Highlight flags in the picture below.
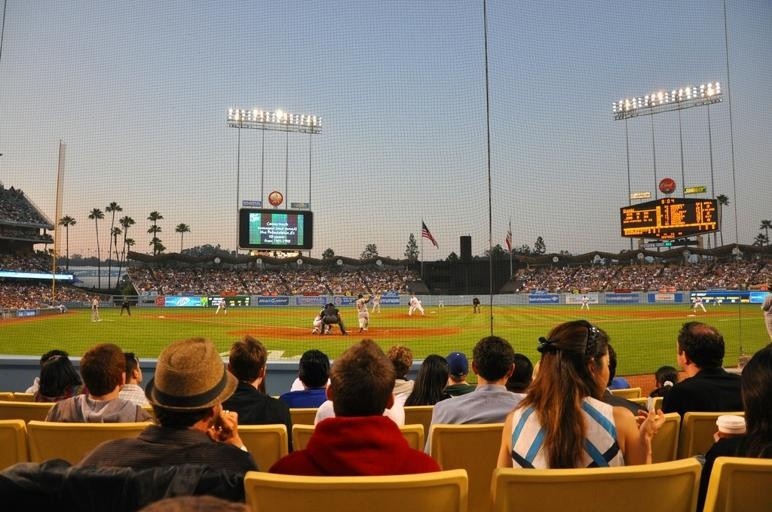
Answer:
[505,231,513,252]
[423,222,440,252]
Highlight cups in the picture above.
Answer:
[714,415,747,439]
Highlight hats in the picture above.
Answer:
[145,337,239,411]
[443,350,469,377]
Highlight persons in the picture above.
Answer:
[473,296,481,313]
[605,322,745,413]
[512,261,771,295]
[696,344,770,510]
[408,296,425,317]
[713,296,721,306]
[424,335,535,456]
[78,337,259,471]
[312,294,380,336]
[216,299,226,315]
[692,296,707,313]
[202,295,208,308]
[125,256,414,296]
[439,297,444,308]
[490,320,666,506]
[267,339,442,475]
[24,292,131,321]
[388,347,474,406]
[0,182,113,314]
[581,296,589,310]
[24,350,151,408]
[223,336,405,429]
